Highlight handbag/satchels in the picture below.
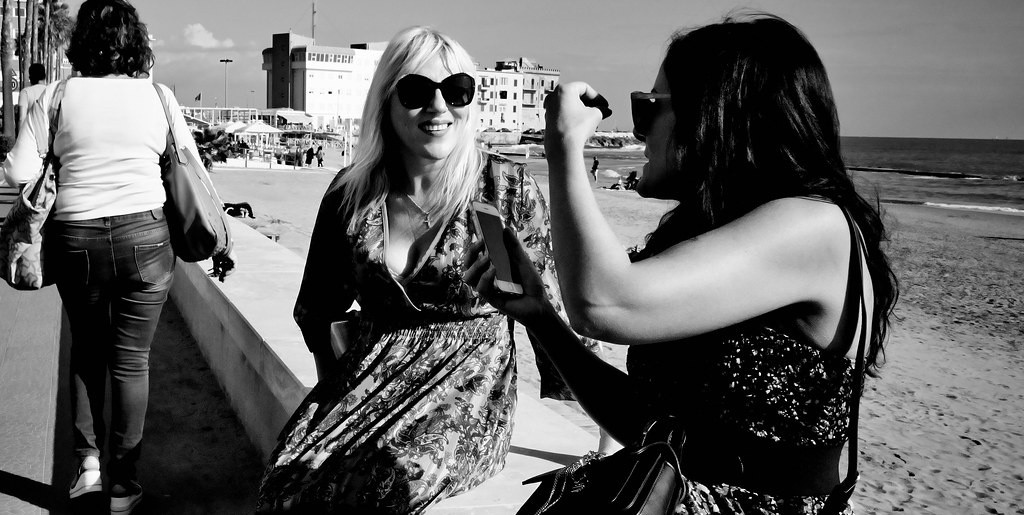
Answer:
[515,432,686,515]
[152,82,229,264]
[0,76,68,292]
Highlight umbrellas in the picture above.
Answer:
[214,120,283,155]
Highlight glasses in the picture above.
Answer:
[631,91,673,141]
[394,72,480,110]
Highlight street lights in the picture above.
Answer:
[220,59,234,109]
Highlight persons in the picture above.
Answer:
[199,137,249,172]
[592,156,599,183]
[294,146,304,169]
[305,146,327,168]
[1,0,237,515]
[17,62,48,129]
[460,15,904,515]
[626,170,637,190]
[254,24,602,515]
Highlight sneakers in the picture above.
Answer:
[110,481,143,515]
[70,455,102,499]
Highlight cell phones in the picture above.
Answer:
[469,200,525,297]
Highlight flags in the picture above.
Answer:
[195,93,201,102]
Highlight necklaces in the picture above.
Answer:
[404,194,441,230]
